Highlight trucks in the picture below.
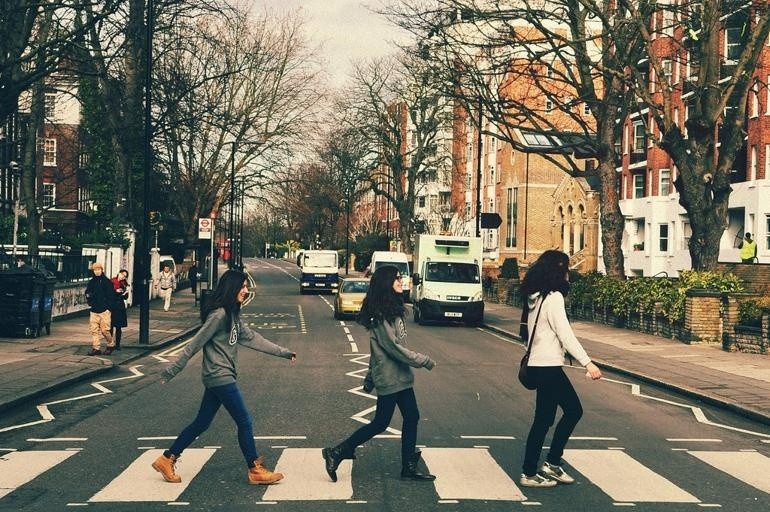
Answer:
[411,234,485,326]
[297,250,340,294]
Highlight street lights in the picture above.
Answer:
[10,161,21,265]
[445,79,484,238]
[221,139,265,270]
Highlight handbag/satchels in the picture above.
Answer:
[518,351,537,390]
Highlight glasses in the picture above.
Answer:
[396,275,402,281]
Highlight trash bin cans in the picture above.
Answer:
[41,272,56,335]
[0,265,46,338]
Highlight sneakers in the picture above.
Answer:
[104,346,116,354]
[520,473,557,487]
[88,349,102,356]
[539,461,574,484]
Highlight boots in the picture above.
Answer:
[152,450,182,482]
[401,451,436,481]
[322,441,356,482]
[248,460,284,485]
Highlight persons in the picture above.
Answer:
[84,263,117,356]
[738,232,757,264]
[633,244,641,251]
[188,262,198,293]
[154,265,176,312]
[322,263,436,481]
[152,269,297,485]
[520,251,602,487]
[108,269,130,351]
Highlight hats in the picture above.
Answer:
[92,263,102,269]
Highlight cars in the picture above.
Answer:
[335,278,372,320]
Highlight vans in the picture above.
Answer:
[369,251,410,303]
